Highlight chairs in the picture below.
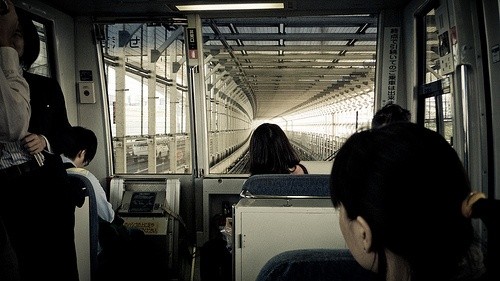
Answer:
[256,248,373,281]
[64,174,98,281]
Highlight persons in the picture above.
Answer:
[0,0,144,281]
[329,119,500,281]
[248,122,308,175]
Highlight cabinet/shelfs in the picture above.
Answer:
[233,198,351,281]
